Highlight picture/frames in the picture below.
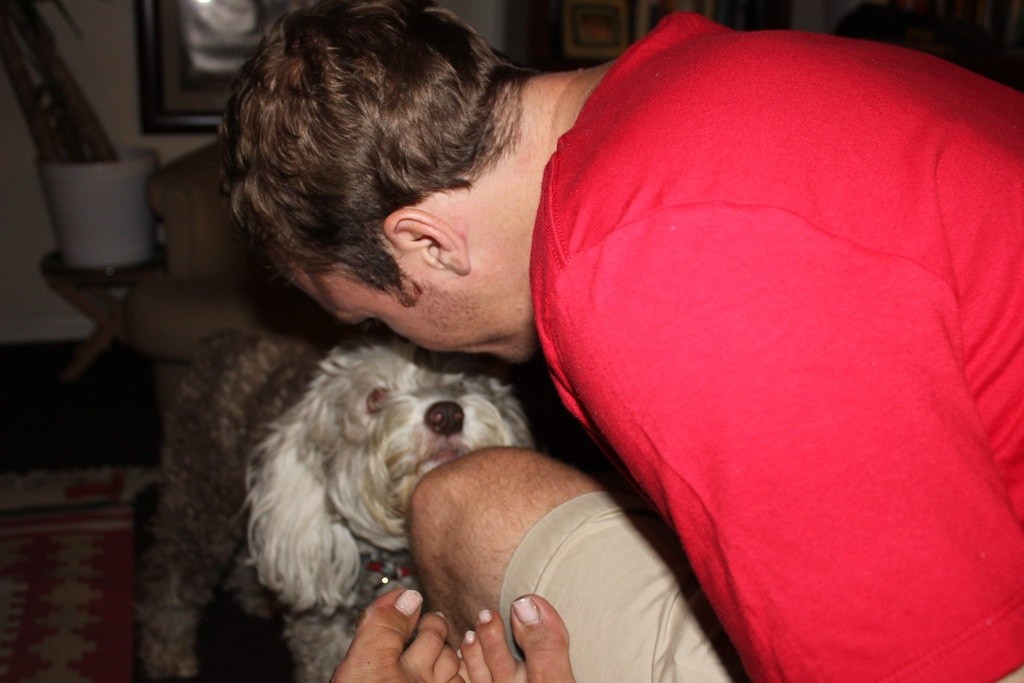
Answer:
[525,0,793,72]
[131,0,288,137]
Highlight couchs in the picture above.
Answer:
[120,143,509,416]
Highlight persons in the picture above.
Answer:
[219,0,1024,683]
[329,586,573,683]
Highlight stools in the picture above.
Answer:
[40,242,170,382]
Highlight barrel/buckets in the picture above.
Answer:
[37,142,159,270]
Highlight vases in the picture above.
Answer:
[34,140,160,269]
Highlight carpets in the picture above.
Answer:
[0,457,154,683]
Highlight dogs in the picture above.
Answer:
[131,321,537,683]
